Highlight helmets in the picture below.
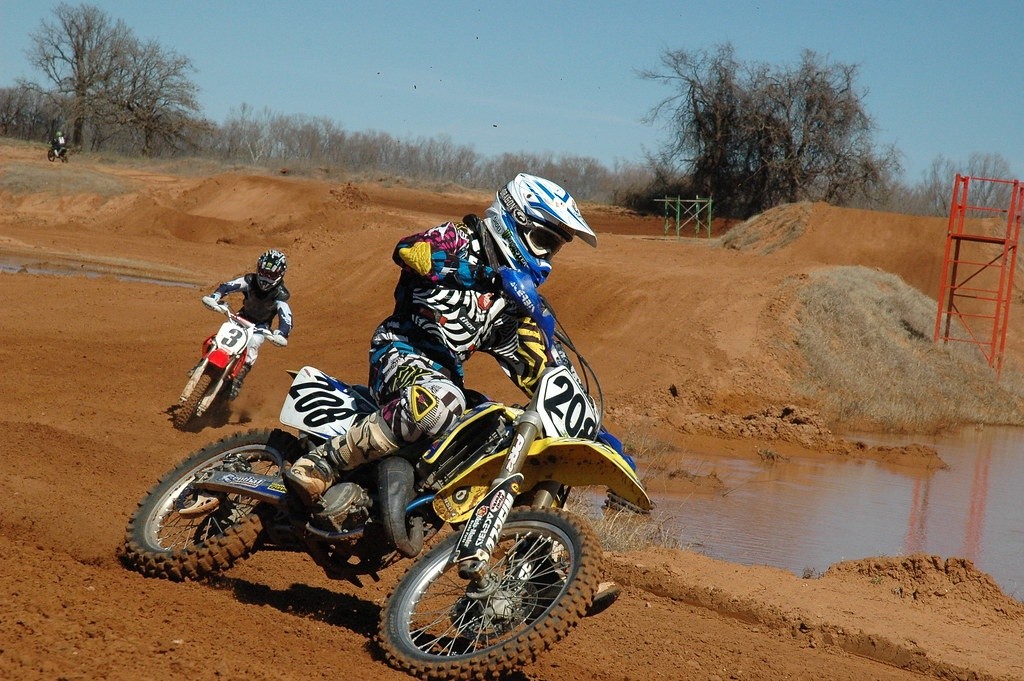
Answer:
[56,132,62,136]
[485,173,597,288]
[257,249,287,290]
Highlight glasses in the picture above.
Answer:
[501,187,568,263]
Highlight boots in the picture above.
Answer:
[286,409,401,501]
[187,334,218,378]
[223,362,251,401]
[520,500,621,624]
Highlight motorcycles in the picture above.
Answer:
[46,139,69,165]
[121,269,655,681]
[173,296,288,432]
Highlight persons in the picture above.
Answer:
[48,131,66,158]
[286,173,610,505]
[186,249,295,401]
